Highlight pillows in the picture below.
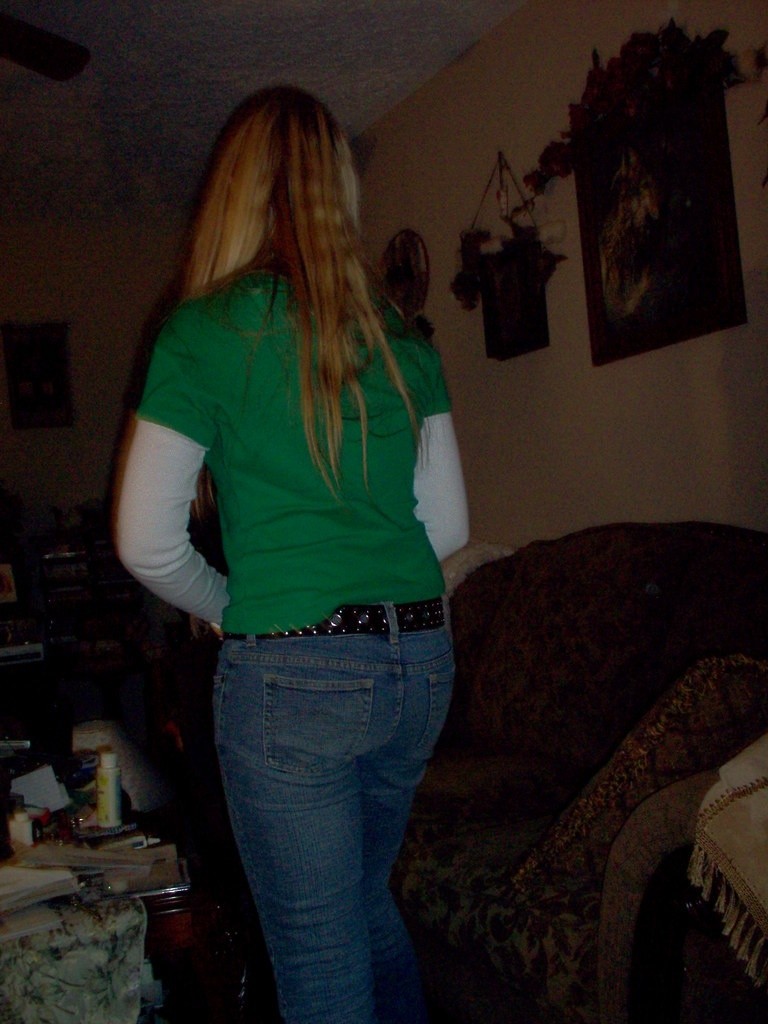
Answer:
[509,649,768,895]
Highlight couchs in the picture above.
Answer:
[388,520,768,1024]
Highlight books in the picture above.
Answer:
[1,840,195,946]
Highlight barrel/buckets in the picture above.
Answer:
[142,887,210,981]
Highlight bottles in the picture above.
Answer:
[97,751,122,828]
[9,809,34,846]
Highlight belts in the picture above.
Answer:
[222,598,445,641]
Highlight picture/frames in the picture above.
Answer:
[567,84,749,365]
[478,247,553,359]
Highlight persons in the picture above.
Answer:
[112,81,475,1024]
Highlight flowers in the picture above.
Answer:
[522,8,748,195]
